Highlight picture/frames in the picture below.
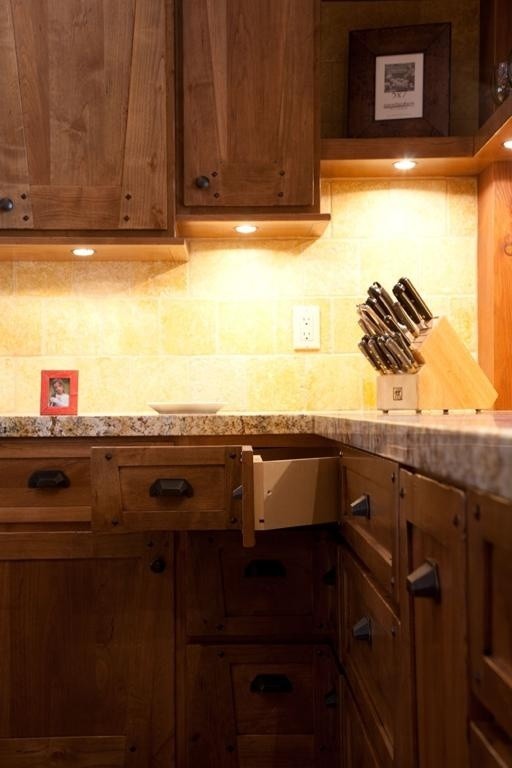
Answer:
[343,21,453,143]
[39,370,78,416]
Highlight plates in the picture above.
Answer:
[146,400,226,415]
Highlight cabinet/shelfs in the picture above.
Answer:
[1,0,191,263]
[177,1,332,243]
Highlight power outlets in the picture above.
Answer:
[292,304,321,351]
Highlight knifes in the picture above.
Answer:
[355,276,434,374]
